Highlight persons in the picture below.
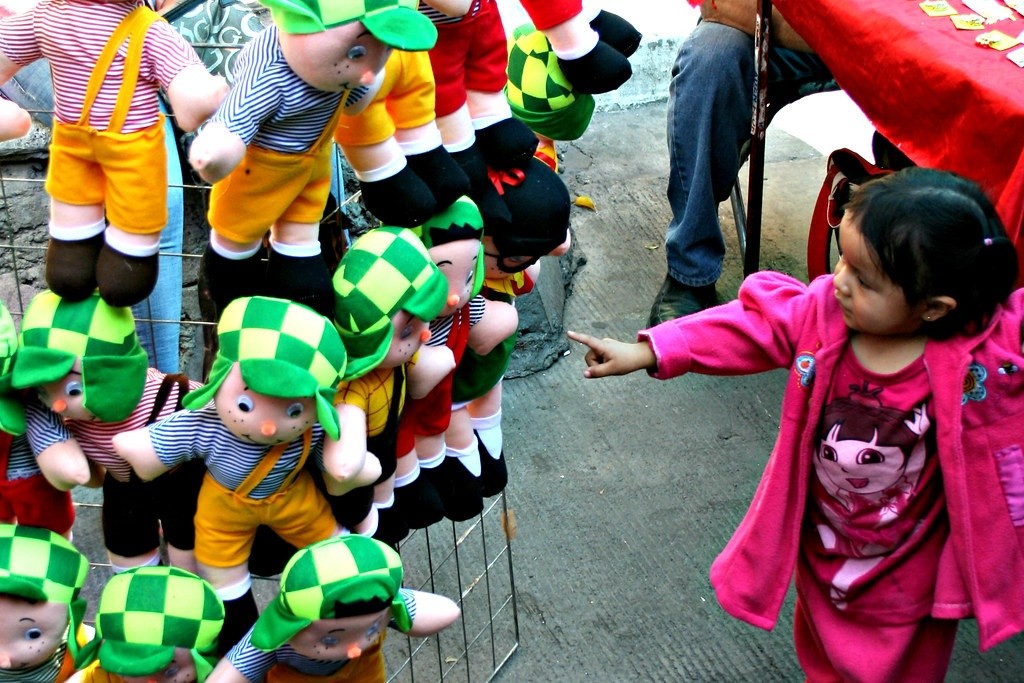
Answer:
[568,168,1024,683]
[646,0,910,328]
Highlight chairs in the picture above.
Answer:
[685,0,842,262]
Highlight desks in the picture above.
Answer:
[744,0,1024,278]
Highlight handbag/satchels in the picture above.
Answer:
[807,148,895,283]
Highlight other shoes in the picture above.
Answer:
[646,273,721,329]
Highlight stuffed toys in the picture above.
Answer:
[0,0,645,683]
